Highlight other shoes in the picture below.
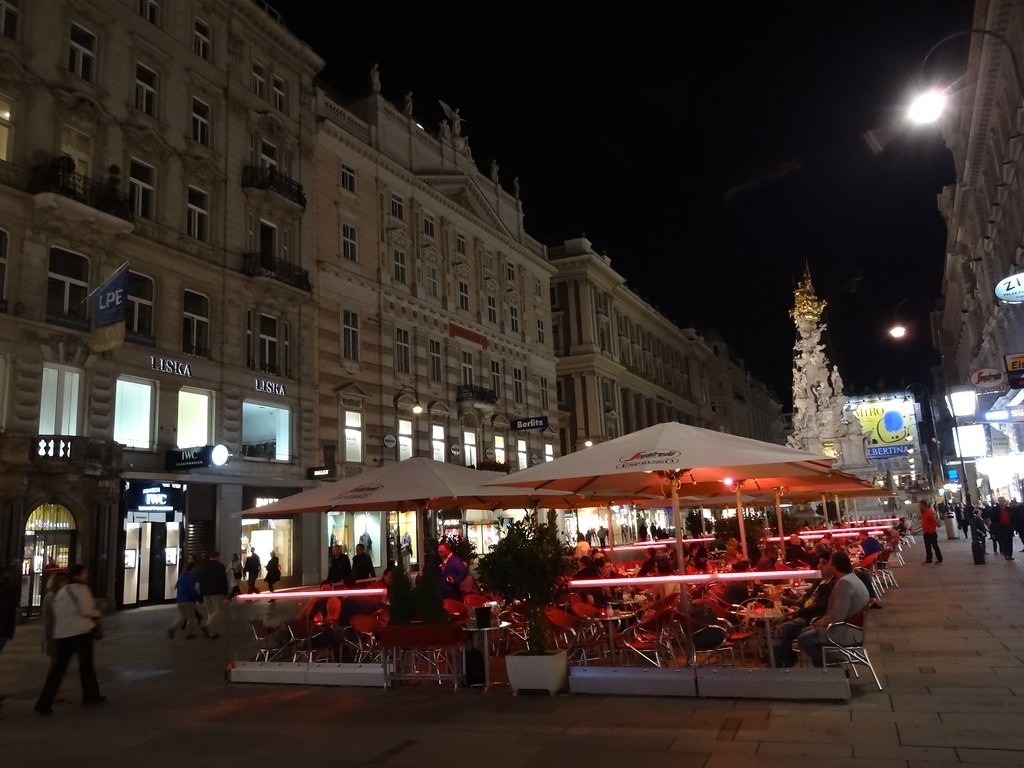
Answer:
[83,696,106,702]
[166,628,175,639]
[185,634,196,639]
[1005,556,1015,560]
[995,551,998,555]
[221,598,229,603]
[922,561,932,563]
[1019,549,1024,552]
[35,703,54,713]
[935,558,943,563]
[984,551,989,554]
[199,625,221,640]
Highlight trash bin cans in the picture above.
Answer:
[944,511,959,539]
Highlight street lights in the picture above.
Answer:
[906,423,941,526]
[572,428,592,533]
[902,382,948,506]
[395,386,422,570]
[889,297,972,508]
[907,27,1024,124]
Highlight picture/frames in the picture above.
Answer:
[124,549,137,569]
[165,546,177,566]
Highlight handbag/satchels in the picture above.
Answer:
[234,572,242,579]
[92,619,103,640]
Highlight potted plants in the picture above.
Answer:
[471,495,578,697]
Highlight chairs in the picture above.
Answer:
[250,526,916,691]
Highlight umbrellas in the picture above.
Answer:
[232,456,586,574]
[583,468,899,588]
[478,421,839,667]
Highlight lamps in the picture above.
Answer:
[571,428,593,447]
[393,386,422,415]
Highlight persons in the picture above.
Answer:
[34,557,105,716]
[167,552,227,641]
[359,531,372,554]
[560,510,912,640]
[0,568,17,702]
[768,551,871,678]
[919,497,1024,564]
[786,262,863,450]
[295,543,469,663]
[370,63,519,200]
[222,547,279,604]
[401,531,412,546]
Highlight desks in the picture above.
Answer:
[609,599,641,633]
[774,582,813,591]
[594,611,635,666]
[462,622,514,694]
[736,608,795,667]
[371,617,470,691]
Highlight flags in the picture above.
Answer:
[91,258,130,352]
